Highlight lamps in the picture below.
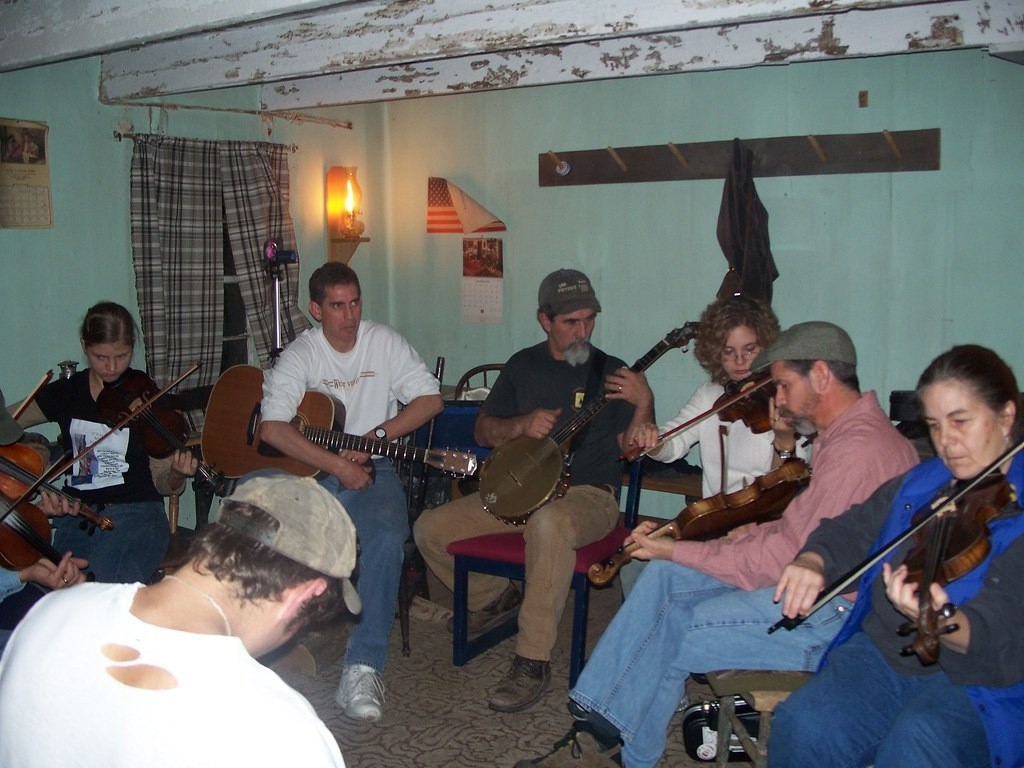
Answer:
[326,165,370,266]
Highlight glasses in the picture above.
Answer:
[721,351,760,361]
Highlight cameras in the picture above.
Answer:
[263,238,297,267]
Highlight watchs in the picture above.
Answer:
[373,426,388,440]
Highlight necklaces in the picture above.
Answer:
[158,571,232,636]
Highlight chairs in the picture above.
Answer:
[396,355,445,657]
[447,456,643,690]
[707,670,813,768]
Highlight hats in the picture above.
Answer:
[0,389,23,445]
[750,321,855,373]
[538,268,601,316]
[214,474,363,615]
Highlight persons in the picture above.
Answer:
[6,302,205,583]
[0,490,87,604]
[259,263,445,723]
[506,321,921,768]
[767,343,1024,768]
[0,475,363,768]
[413,269,657,715]
[620,295,813,603]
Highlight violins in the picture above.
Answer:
[95,369,228,497]
[0,443,114,532]
[893,466,1015,667]
[0,496,97,584]
[587,457,814,586]
[712,373,818,447]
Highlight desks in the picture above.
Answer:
[451,462,700,501]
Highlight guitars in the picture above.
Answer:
[479,320,704,526]
[201,365,480,481]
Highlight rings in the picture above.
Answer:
[64,579,68,583]
[773,418,780,422]
[619,386,623,392]
[351,457,359,462]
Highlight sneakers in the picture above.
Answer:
[516,723,622,768]
[447,581,522,635]
[334,664,386,722]
[488,657,550,711]
